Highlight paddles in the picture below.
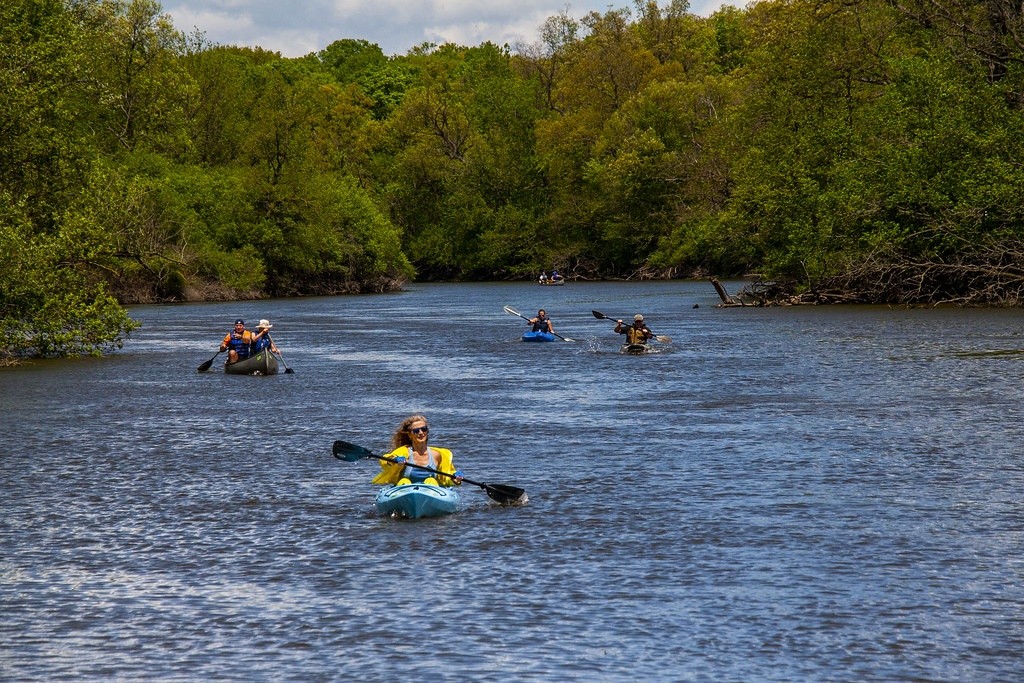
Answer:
[265,328,295,374]
[588,308,672,343]
[198,346,227,372]
[501,302,575,343]
[332,438,530,509]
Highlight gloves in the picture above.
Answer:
[394,457,406,464]
[453,471,465,480]
[551,331,554,333]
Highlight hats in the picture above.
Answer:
[235,319,244,325]
[633,313,644,321]
[553,271,557,276]
[255,319,273,328]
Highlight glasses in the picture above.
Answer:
[411,426,427,434]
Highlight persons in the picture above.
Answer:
[613,313,654,348]
[527,309,555,334]
[252,319,280,355]
[551,270,563,282]
[371,414,465,488]
[540,271,549,281]
[220,318,252,364]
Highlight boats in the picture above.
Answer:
[620,343,652,354]
[539,278,565,286]
[224,347,280,375]
[522,331,554,342]
[375,482,461,518]
